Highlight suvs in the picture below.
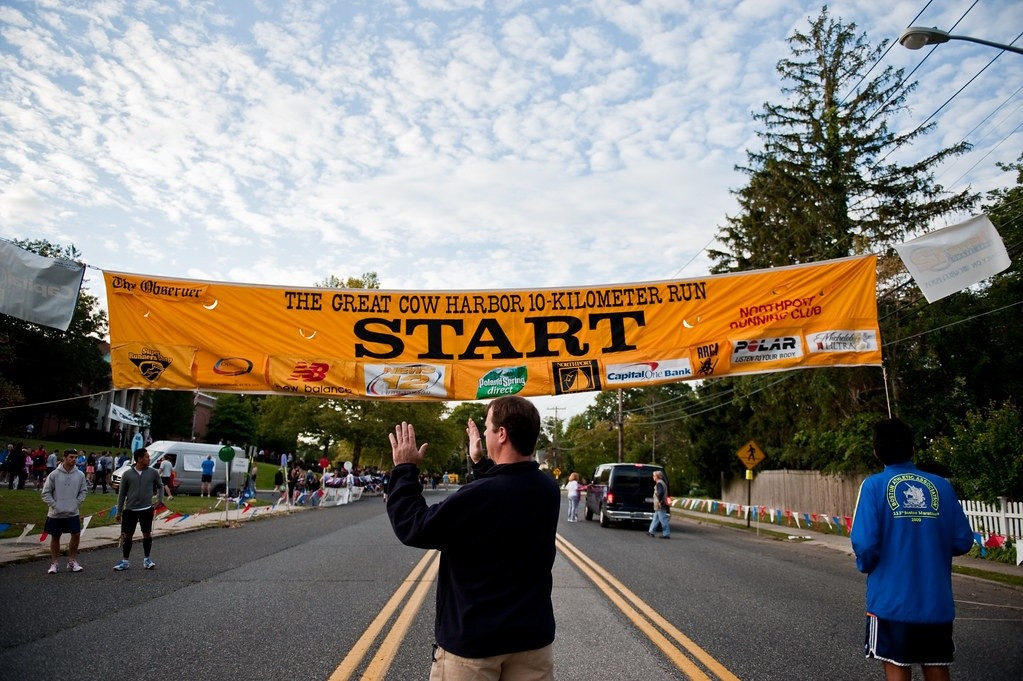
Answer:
[583,463,670,529]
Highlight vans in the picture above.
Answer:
[109,441,246,497]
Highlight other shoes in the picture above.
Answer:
[646,533,655,537]
[660,535,671,539]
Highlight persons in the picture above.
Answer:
[646,470,672,539]
[40,449,88,574]
[564,473,578,523]
[852,417,974,681]
[575,477,593,519]
[113,449,164,570]
[386,395,563,681]
[0,436,453,508]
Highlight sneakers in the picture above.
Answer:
[114,560,129,571]
[48,563,60,573]
[67,561,83,571]
[143,559,155,569]
